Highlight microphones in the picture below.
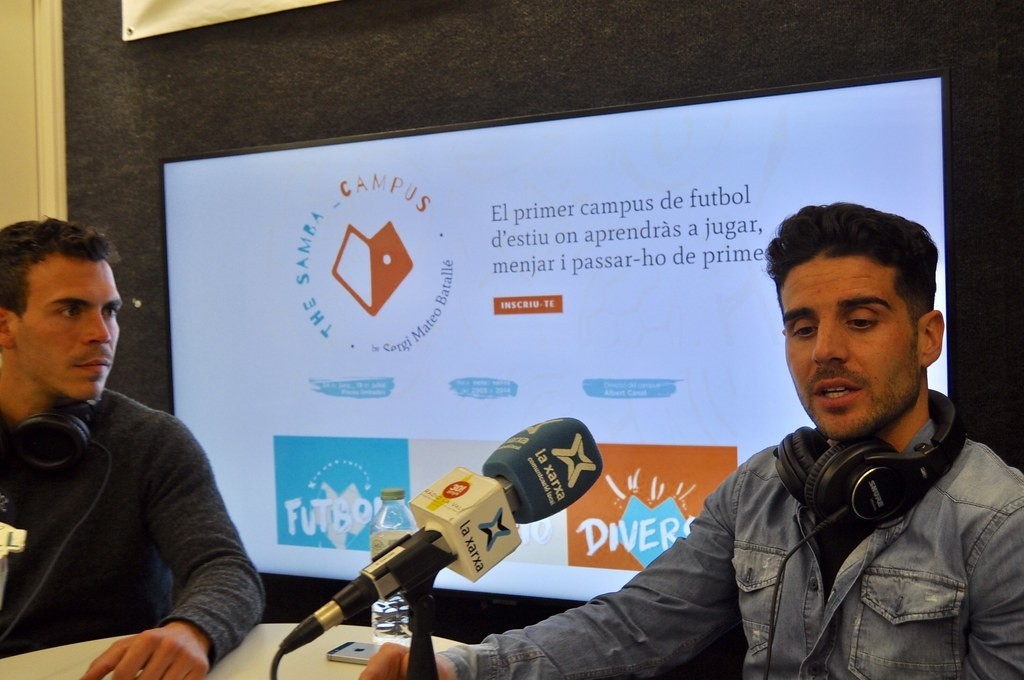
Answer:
[280,418,603,654]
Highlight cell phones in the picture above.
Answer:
[327,642,382,664]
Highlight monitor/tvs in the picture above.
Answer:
[157,66,951,613]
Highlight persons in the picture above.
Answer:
[0,218,265,680]
[359,203,1024,680]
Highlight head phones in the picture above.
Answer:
[0,398,102,482]
[772,388,967,534]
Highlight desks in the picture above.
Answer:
[0,622,464,680]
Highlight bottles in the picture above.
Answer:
[368,488,414,651]
